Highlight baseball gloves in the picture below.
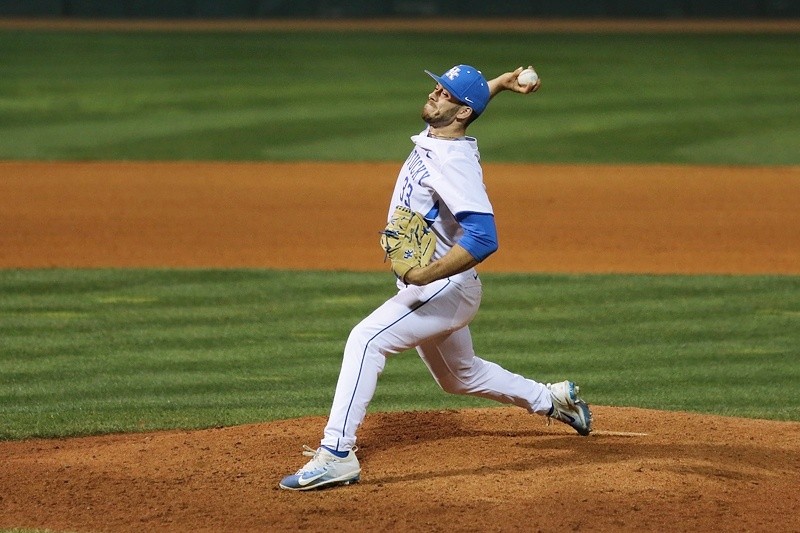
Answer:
[378,203,438,289]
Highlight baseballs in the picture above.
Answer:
[517,68,539,87]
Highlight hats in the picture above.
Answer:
[424,64,491,116]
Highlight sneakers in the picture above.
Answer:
[546,379,593,436]
[279,444,362,490]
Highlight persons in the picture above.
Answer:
[278,65,594,492]
